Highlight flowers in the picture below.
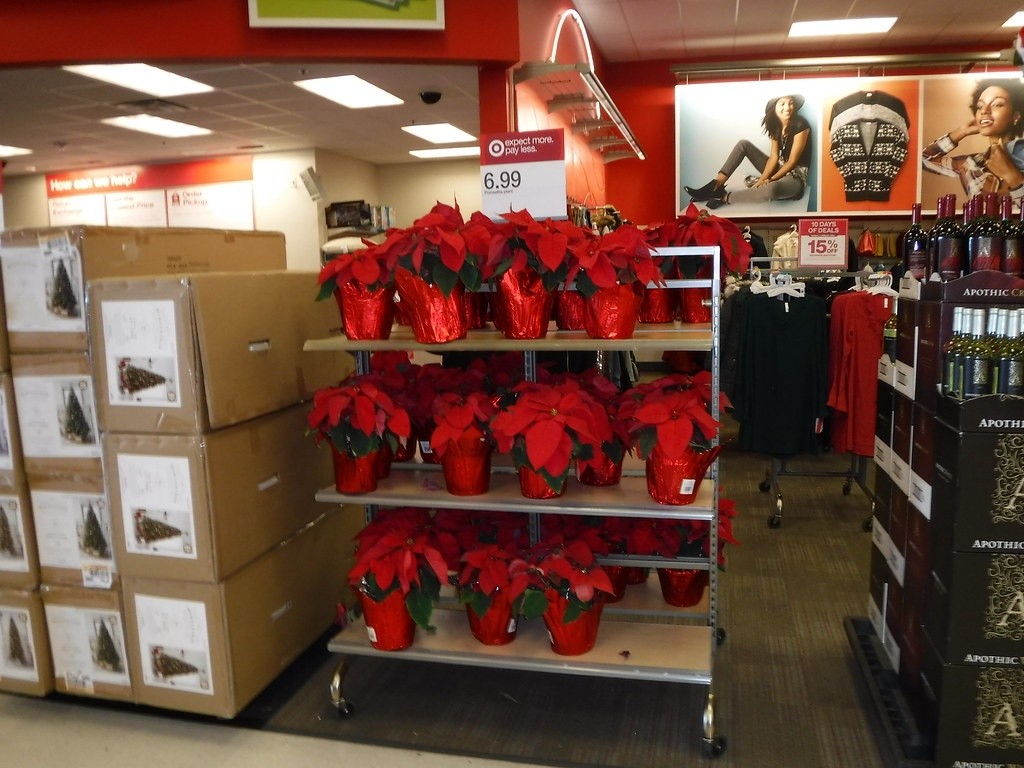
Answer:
[307,192,743,628]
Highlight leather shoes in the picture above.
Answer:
[684,180,728,209]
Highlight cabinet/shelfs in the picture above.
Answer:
[301,244,730,758]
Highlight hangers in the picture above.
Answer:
[731,224,899,297]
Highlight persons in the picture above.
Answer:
[684,94,810,208]
[923,78,1024,210]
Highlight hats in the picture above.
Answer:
[765,89,805,112]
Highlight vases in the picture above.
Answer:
[331,268,709,656]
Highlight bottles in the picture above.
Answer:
[940,304,1024,400]
[903,188,1024,282]
[883,294,900,367]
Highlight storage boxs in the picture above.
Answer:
[0,199,395,719]
[867,270,1024,768]
